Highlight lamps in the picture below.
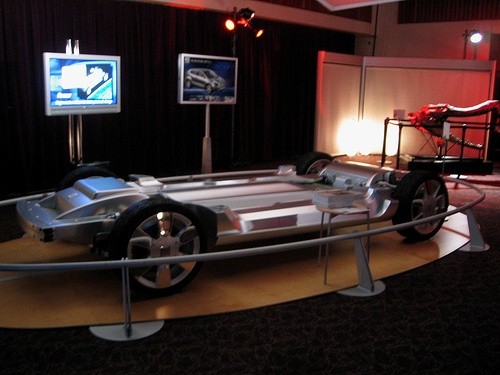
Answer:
[224,7,264,37]
[463,29,482,59]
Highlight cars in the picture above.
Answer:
[185,68,225,95]
